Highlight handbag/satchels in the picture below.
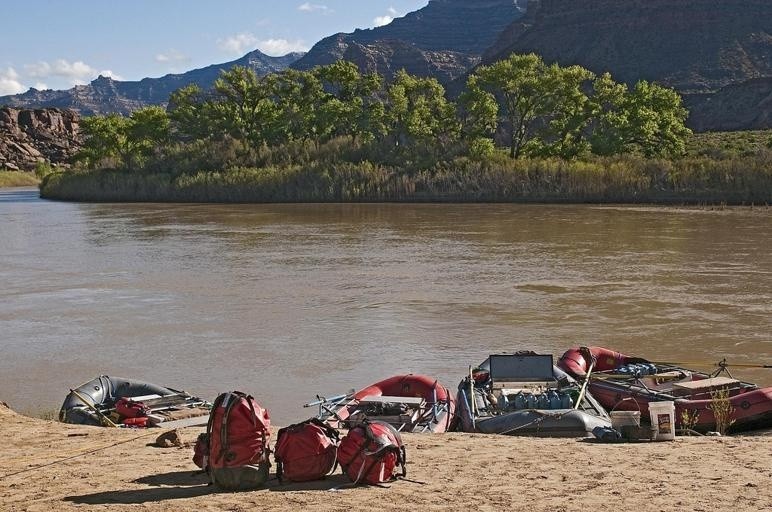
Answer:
[338,421,402,484]
[274,418,338,482]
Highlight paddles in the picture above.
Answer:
[469,365,476,430]
[303,389,355,408]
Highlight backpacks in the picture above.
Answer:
[204,391,270,491]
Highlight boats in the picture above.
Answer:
[459,352,612,438]
[316,373,455,435]
[58,374,212,430]
[558,346,772,432]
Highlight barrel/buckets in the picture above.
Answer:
[609,396,641,440]
[649,400,676,441]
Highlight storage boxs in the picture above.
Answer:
[673,377,740,399]
